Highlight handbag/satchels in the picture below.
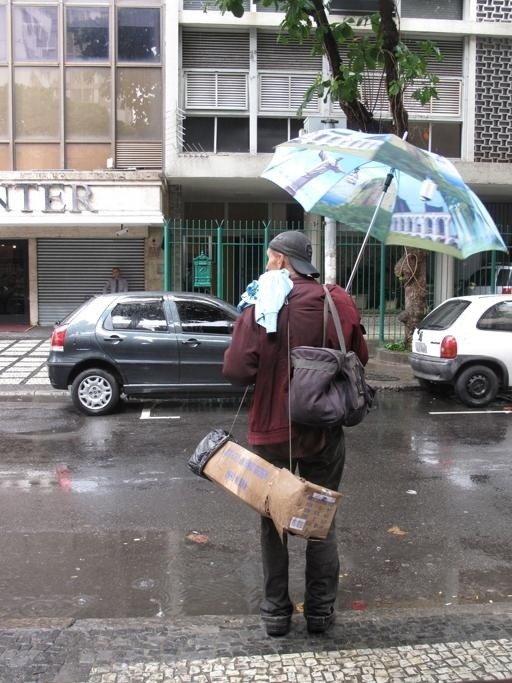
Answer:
[288,279,368,427]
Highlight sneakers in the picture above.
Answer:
[262,613,335,636]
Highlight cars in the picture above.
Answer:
[407,262,512,408]
[46,291,255,416]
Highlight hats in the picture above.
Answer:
[268,230,321,282]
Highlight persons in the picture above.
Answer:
[222,228,370,639]
[102,266,129,294]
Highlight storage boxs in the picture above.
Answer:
[203,441,343,545]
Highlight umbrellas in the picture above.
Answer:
[260,125,512,297]
